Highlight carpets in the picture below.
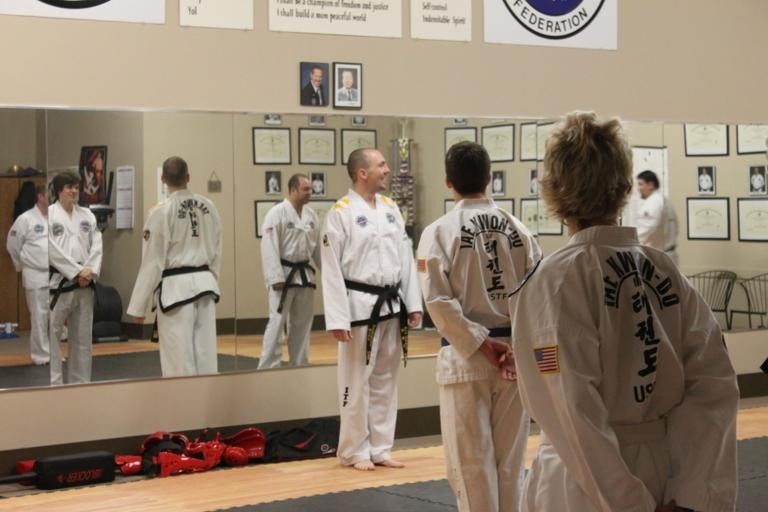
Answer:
[0,349,289,389]
[201,435,767,512]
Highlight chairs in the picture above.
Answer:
[727,273,766,330]
[685,269,735,330]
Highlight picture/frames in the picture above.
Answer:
[242,118,382,241]
[677,123,765,244]
[331,62,363,110]
[436,120,566,240]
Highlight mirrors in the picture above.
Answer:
[0,107,765,393]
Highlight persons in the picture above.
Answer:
[318,144,426,471]
[312,174,323,194]
[751,168,765,193]
[493,172,504,194]
[635,171,680,271]
[336,68,357,101]
[6,185,66,367]
[300,65,324,106]
[508,108,740,511]
[414,141,547,512]
[530,171,536,192]
[256,174,319,370]
[47,170,104,389]
[698,168,713,192]
[124,154,224,377]
[268,172,279,193]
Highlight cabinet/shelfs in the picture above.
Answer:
[0,169,46,331]
